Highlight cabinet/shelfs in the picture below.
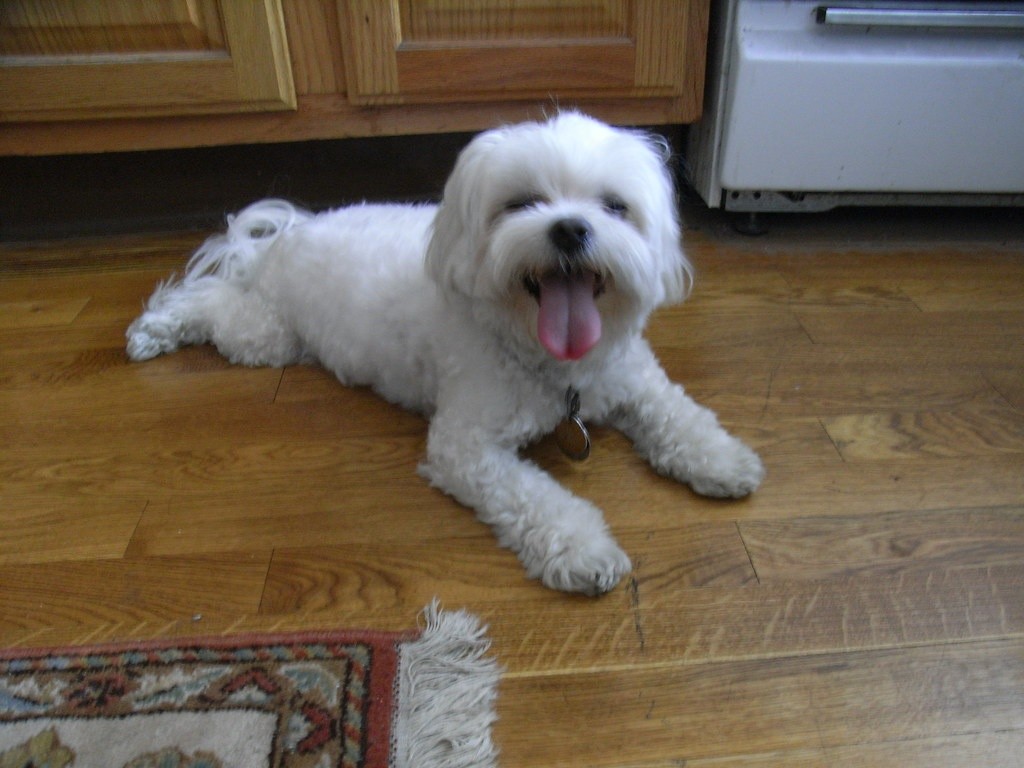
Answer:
[0,0,712,157]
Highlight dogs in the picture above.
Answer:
[125,91,763,598]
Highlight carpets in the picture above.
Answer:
[0,590,507,768]
